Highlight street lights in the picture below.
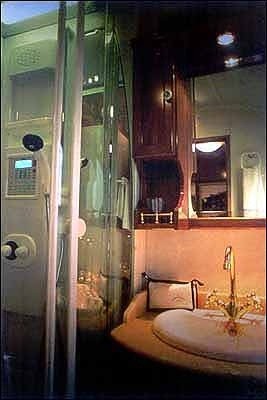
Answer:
[141,272,204,311]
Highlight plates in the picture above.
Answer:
[132,63,189,229]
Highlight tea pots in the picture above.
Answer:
[189,51,265,228]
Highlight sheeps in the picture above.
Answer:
[222,245,239,319]
